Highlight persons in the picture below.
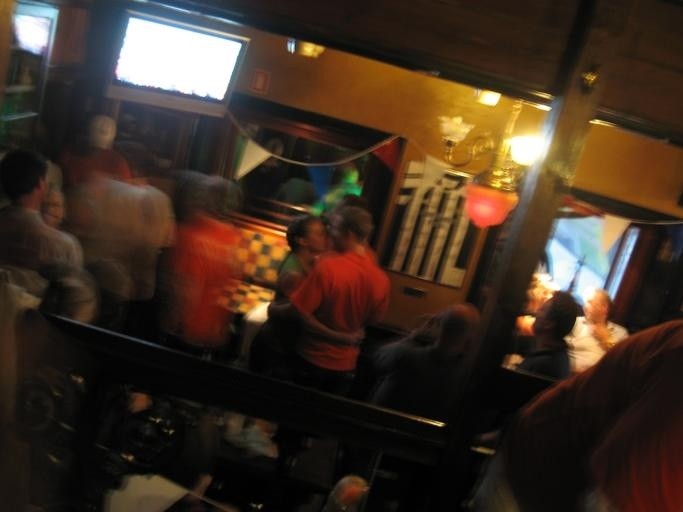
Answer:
[504,317,682,512]
[0,114,632,509]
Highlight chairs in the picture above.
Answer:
[209,215,291,355]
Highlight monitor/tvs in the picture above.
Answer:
[219,108,380,235]
[103,7,251,119]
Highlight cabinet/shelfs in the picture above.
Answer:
[0,1,58,153]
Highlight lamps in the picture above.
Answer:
[439,74,558,230]
